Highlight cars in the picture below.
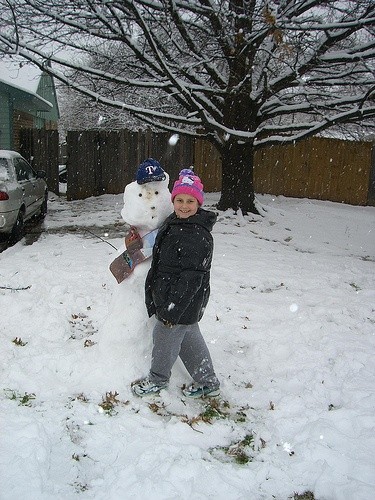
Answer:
[0,149,49,238]
[58,156,67,182]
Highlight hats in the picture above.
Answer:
[171,169,205,205]
[135,159,166,185]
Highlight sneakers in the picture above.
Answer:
[183,382,220,398]
[131,373,171,396]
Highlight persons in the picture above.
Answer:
[125,169,221,399]
